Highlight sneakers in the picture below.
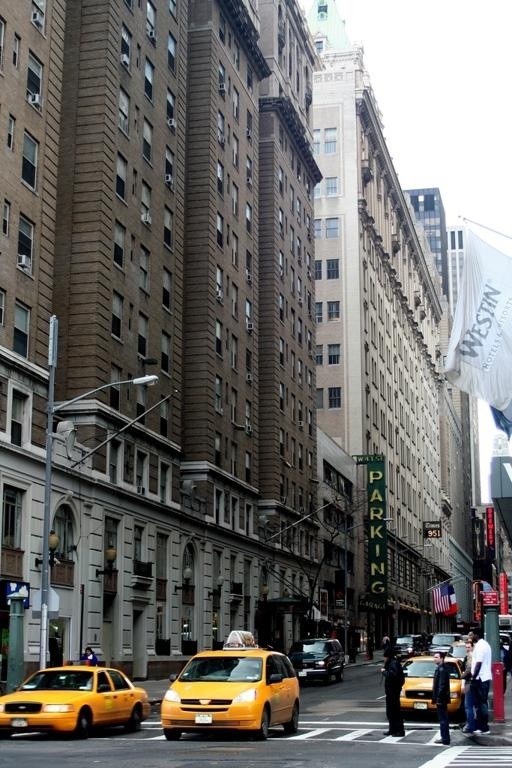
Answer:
[459,723,490,735]
[435,738,450,744]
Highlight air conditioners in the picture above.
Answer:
[146,28,156,40]
[167,117,177,131]
[18,254,30,270]
[219,82,226,93]
[219,135,227,145]
[29,93,43,109]
[32,12,44,28]
[122,54,132,67]
[166,174,173,184]
[141,214,153,227]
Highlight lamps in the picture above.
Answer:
[260,584,269,602]
[34,530,224,598]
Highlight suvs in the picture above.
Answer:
[287,637,346,685]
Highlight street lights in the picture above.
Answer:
[38,312,162,670]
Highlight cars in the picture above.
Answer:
[395,633,462,661]
[399,655,471,721]
[1,664,153,739]
[160,630,301,741]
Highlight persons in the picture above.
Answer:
[80,646,98,666]
[379,647,406,737]
[382,633,391,645]
[457,640,477,733]
[465,627,493,736]
[430,651,450,745]
[498,635,512,695]
[365,636,374,660]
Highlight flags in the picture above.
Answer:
[440,584,458,618]
[442,227,511,438]
[431,583,450,614]
